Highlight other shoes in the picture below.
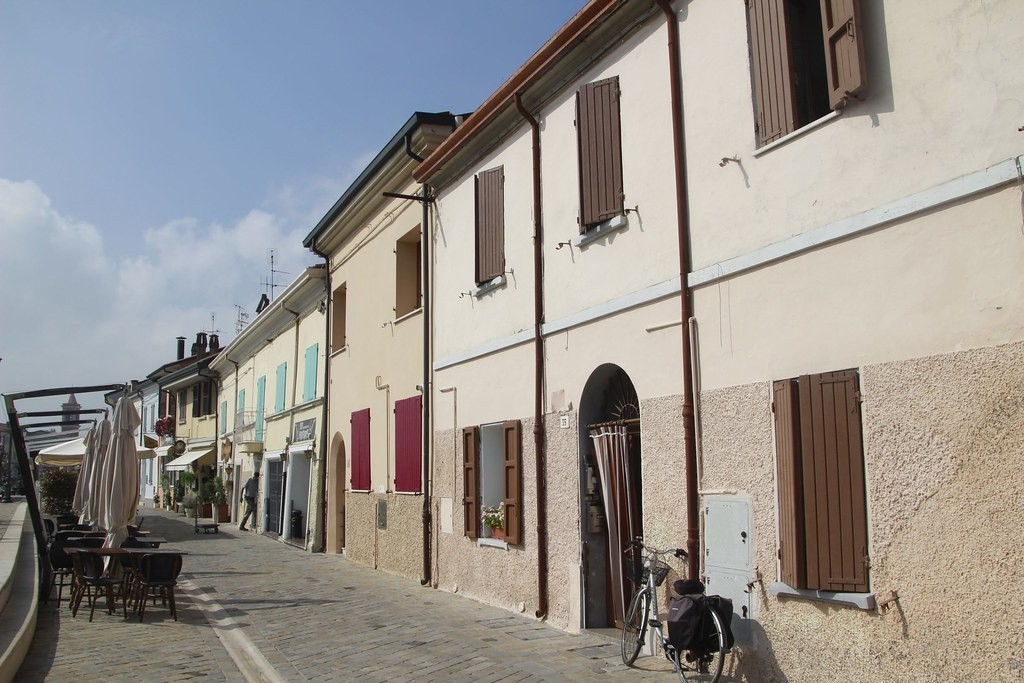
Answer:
[239,526,249,531]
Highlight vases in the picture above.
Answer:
[490,526,505,537]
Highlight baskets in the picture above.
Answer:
[625,555,671,586]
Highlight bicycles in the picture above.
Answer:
[620,535,730,683]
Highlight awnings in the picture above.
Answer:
[156,445,174,456]
[165,447,216,471]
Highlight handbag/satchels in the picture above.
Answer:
[667,594,735,653]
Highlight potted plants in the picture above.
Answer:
[180,489,196,517]
[198,480,213,517]
[165,491,172,511]
[212,477,229,524]
[153,494,159,508]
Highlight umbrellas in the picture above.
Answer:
[35,382,157,579]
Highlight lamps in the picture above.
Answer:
[225,463,234,475]
[279,441,292,461]
[305,438,317,460]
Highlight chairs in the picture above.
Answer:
[44,512,186,623]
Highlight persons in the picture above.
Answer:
[239,472,259,531]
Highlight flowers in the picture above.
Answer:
[479,501,504,527]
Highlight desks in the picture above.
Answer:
[60,530,107,536]
[124,546,189,612]
[125,536,168,548]
[63,548,129,610]
[135,530,151,536]
[66,535,105,548]
